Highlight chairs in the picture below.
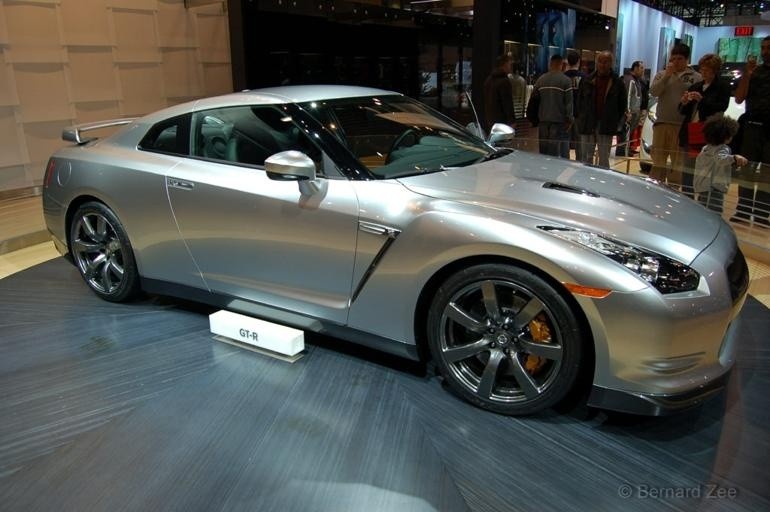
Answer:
[228,115,319,168]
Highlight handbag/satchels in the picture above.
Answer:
[526,87,541,127]
[687,122,707,158]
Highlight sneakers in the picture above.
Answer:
[615,149,639,157]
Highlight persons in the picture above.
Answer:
[728,35,770,230]
[478,55,519,130]
[529,43,754,214]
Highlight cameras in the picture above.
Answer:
[688,92,692,100]
